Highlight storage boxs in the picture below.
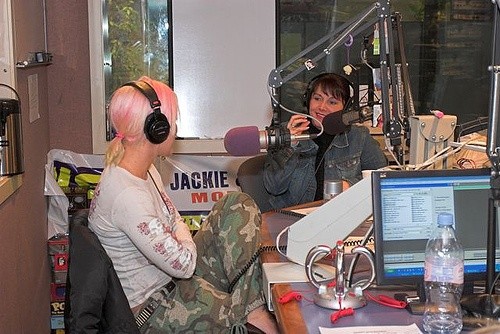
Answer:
[49,238,68,334]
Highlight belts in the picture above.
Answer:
[135,281,175,328]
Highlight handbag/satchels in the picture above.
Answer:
[409,114,457,170]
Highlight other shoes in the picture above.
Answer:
[238,321,265,334]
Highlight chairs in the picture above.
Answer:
[237,154,272,213]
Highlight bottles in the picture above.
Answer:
[422,213,464,334]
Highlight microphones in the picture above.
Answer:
[322,106,371,136]
[223,126,318,156]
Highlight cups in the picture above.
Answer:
[324,180,343,203]
[362,170,377,179]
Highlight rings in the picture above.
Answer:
[291,124,294,128]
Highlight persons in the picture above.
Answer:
[87,76,281,334]
[263,72,388,210]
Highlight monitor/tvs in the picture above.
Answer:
[371,167,500,316]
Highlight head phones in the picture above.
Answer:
[301,72,359,112]
[121,80,170,144]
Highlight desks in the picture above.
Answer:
[259,200,500,334]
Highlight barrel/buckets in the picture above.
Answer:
[0,83,24,175]
